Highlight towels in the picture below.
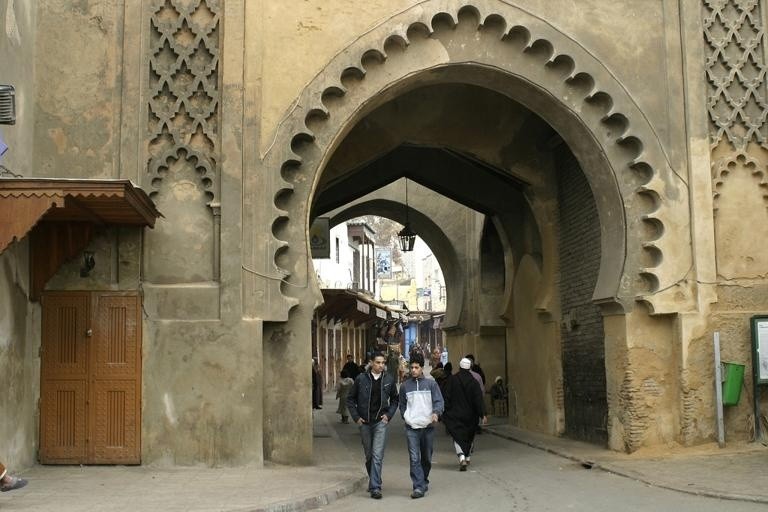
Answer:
[720,361,745,406]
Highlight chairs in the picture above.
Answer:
[410,490,425,498]
[369,490,383,498]
[459,455,472,471]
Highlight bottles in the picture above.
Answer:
[459,357,472,371]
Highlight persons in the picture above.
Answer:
[400,357,444,499]
[442,358,487,472]
[312,341,508,433]
[0,463,28,493]
[348,354,399,500]
